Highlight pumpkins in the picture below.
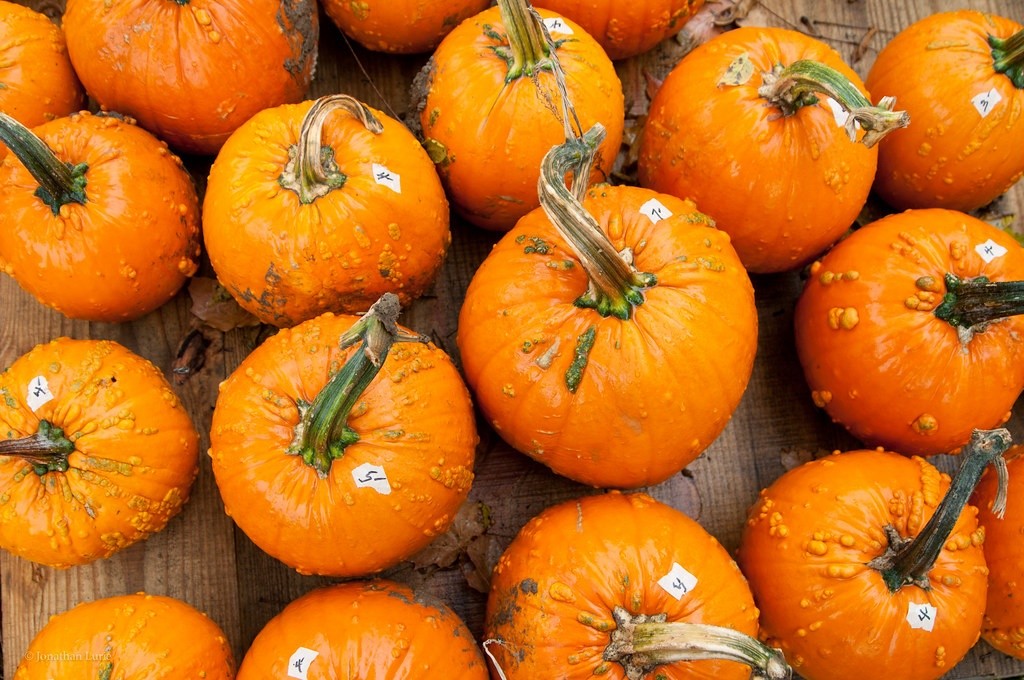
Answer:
[0,0,1024,680]
